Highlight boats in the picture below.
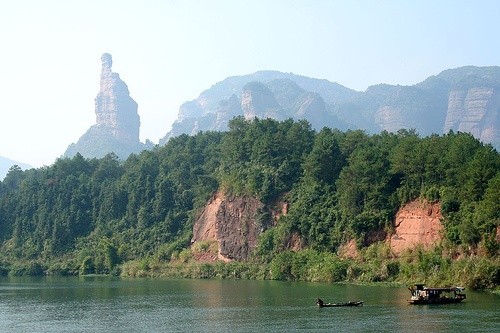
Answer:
[405,282,466,305]
[316,297,364,308]
